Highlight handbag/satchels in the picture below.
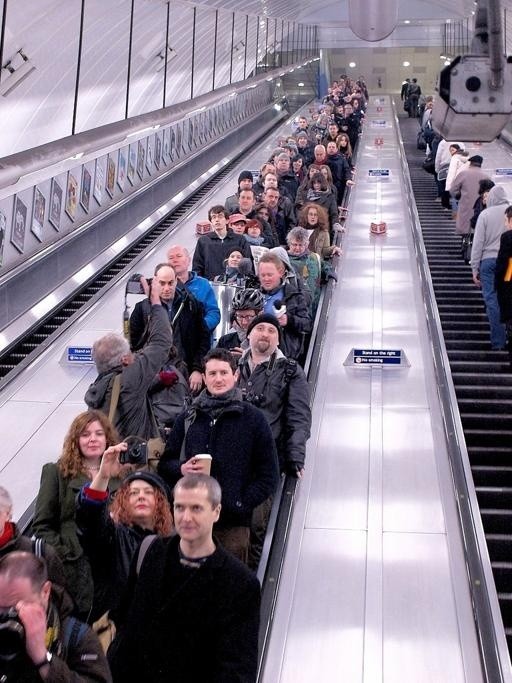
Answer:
[422,154,435,174]
[461,224,471,263]
[416,132,427,150]
[404,101,410,111]
[108,430,166,471]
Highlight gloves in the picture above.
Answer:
[325,271,338,282]
[159,370,178,387]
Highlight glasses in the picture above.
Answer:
[237,314,254,320]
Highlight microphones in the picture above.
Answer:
[272,299,287,331]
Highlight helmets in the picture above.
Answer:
[232,288,264,310]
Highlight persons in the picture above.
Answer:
[83,185,344,474]
[225,75,368,234]
[0,349,278,682]
[401,76,511,372]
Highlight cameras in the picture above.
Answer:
[118,444,148,464]
[126,274,152,294]
[0,607,26,661]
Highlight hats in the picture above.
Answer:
[238,171,253,185]
[228,214,247,225]
[246,314,282,339]
[297,131,308,141]
[468,156,482,163]
[124,470,168,496]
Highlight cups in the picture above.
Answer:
[193,452,212,479]
